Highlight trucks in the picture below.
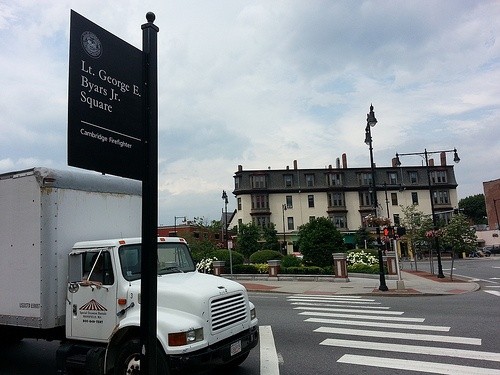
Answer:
[0,165,260,375]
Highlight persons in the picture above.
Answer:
[80,253,109,288]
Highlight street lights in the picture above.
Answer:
[363,101,389,292]
[394,146,461,278]
[222,189,229,250]
[282,203,287,255]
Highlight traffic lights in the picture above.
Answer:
[383,228,390,236]
